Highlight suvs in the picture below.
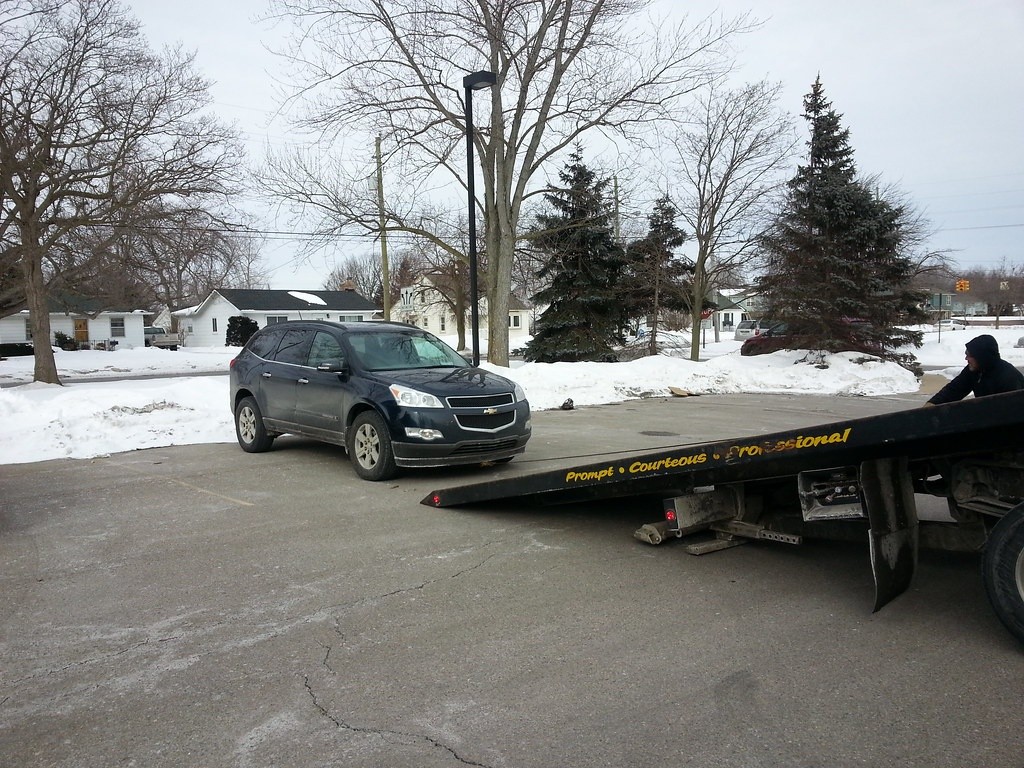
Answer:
[230,320,531,481]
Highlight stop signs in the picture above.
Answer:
[700,308,710,329]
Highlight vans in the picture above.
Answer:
[736,315,881,356]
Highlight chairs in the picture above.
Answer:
[364,336,382,356]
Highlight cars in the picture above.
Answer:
[933,319,966,331]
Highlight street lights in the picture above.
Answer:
[462,70,497,368]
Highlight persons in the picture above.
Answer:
[928,335,1024,405]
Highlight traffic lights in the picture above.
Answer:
[956,280,969,292]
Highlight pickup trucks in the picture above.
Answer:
[144,325,180,351]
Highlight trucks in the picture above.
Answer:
[420,386,1024,644]
